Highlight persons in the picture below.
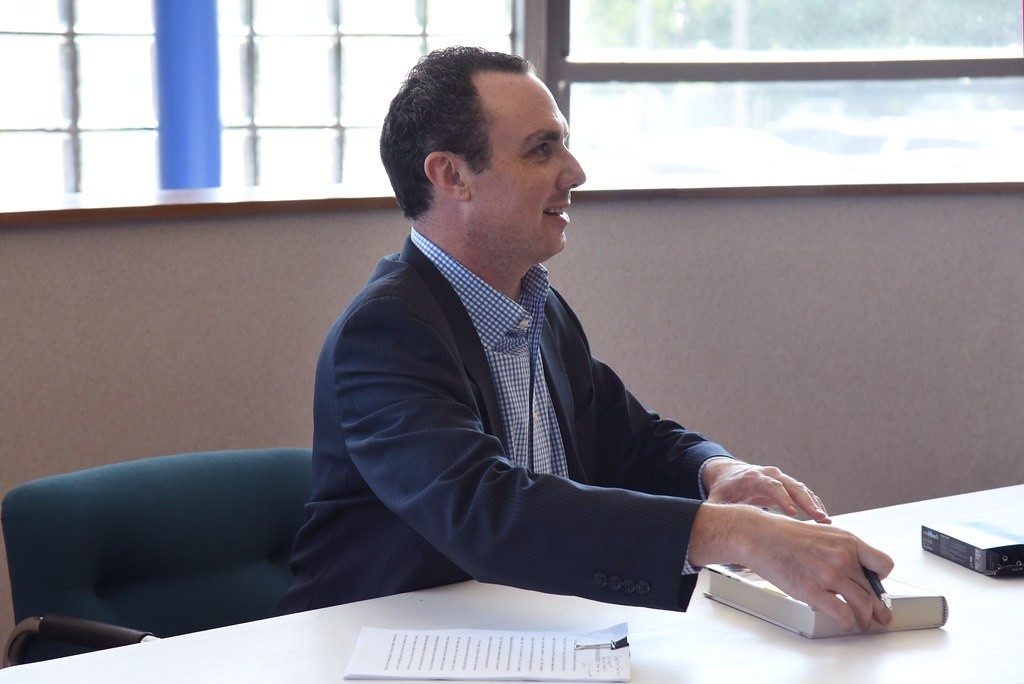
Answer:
[284,45,896,634]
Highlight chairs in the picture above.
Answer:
[1,434,317,668]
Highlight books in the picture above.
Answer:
[702,563,950,641]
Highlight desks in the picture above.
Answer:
[1,481,1023,667]
